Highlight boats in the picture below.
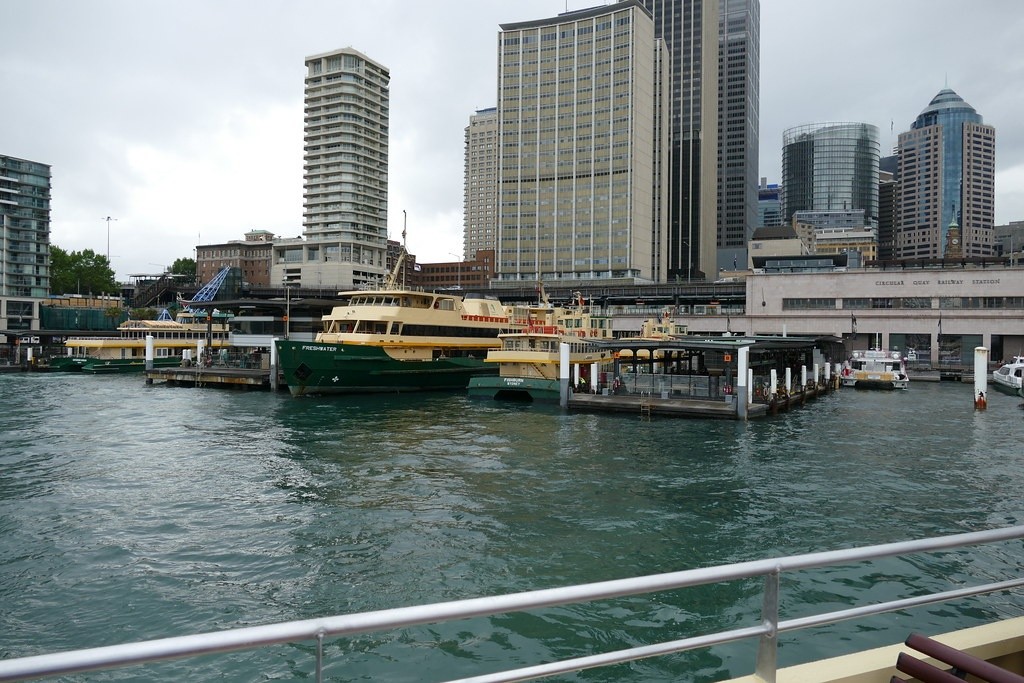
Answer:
[842,350,910,389]
[273,251,528,396]
[47,311,236,374]
[465,250,621,401]
[618,311,689,374]
[992,356,1024,388]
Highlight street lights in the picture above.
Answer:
[448,253,461,290]
[101,216,117,269]
[402,210,406,290]
